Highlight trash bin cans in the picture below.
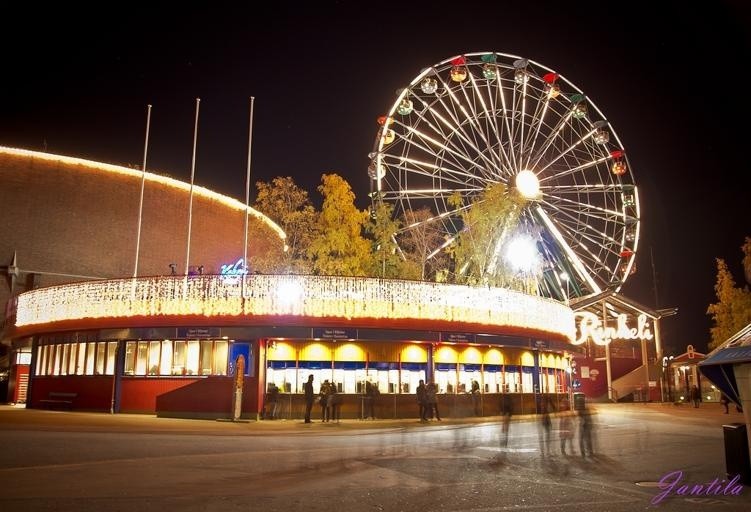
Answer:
[721,423,751,486]
[574,392,585,410]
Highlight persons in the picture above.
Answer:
[498,394,514,437]
[363,380,377,420]
[472,380,481,415]
[446,381,453,393]
[424,377,442,422]
[319,379,331,424]
[467,378,474,393]
[691,384,702,409]
[720,393,732,415]
[534,393,557,459]
[575,397,594,459]
[302,374,314,424]
[330,382,341,424]
[558,400,577,456]
[416,380,429,421]
